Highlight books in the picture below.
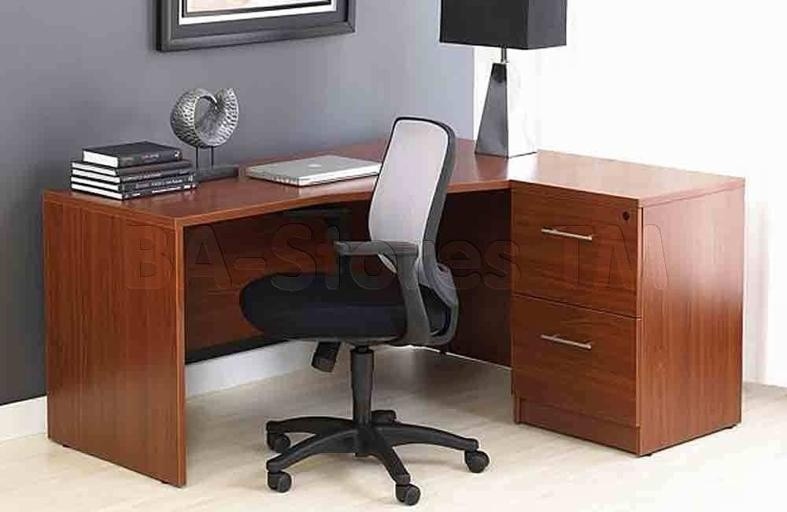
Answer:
[246,151,383,189]
[70,139,199,201]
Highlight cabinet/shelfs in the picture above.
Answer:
[40,135,744,488]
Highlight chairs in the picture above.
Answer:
[239,117,489,505]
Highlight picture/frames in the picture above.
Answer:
[153,0,359,52]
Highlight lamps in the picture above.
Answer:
[439,0,567,160]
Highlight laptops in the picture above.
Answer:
[244,154,382,187]
